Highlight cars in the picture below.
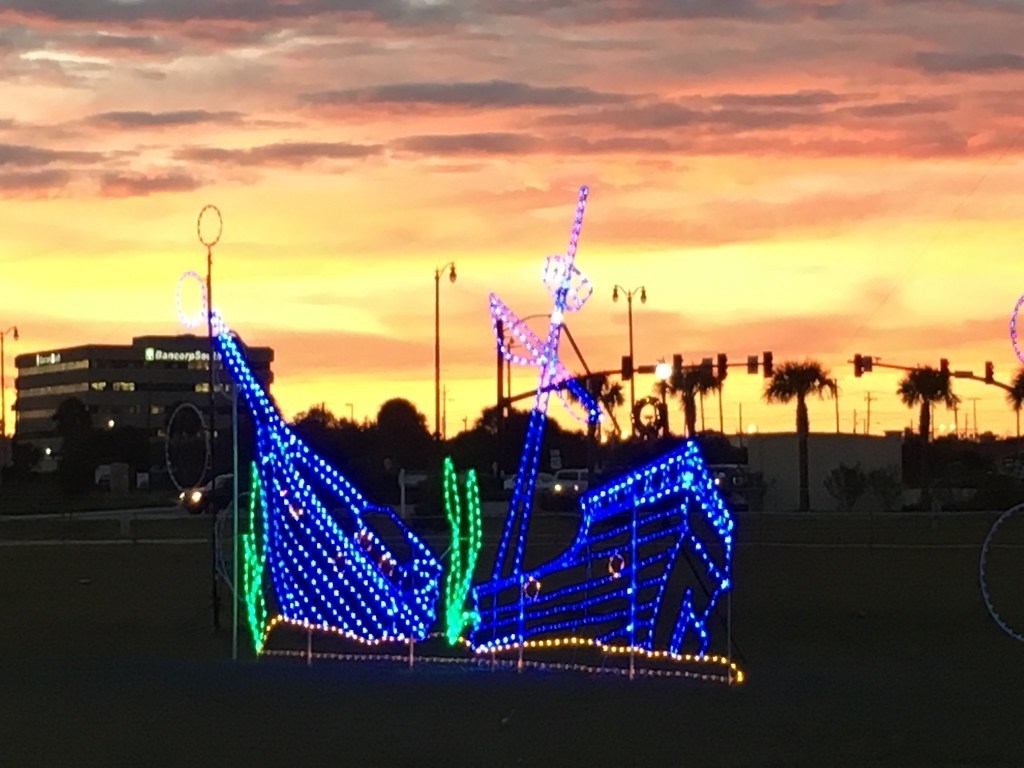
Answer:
[504,471,579,509]
[178,473,250,517]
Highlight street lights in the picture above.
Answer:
[0,325,19,465]
[434,260,457,440]
[612,282,648,434]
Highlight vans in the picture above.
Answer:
[553,469,602,494]
